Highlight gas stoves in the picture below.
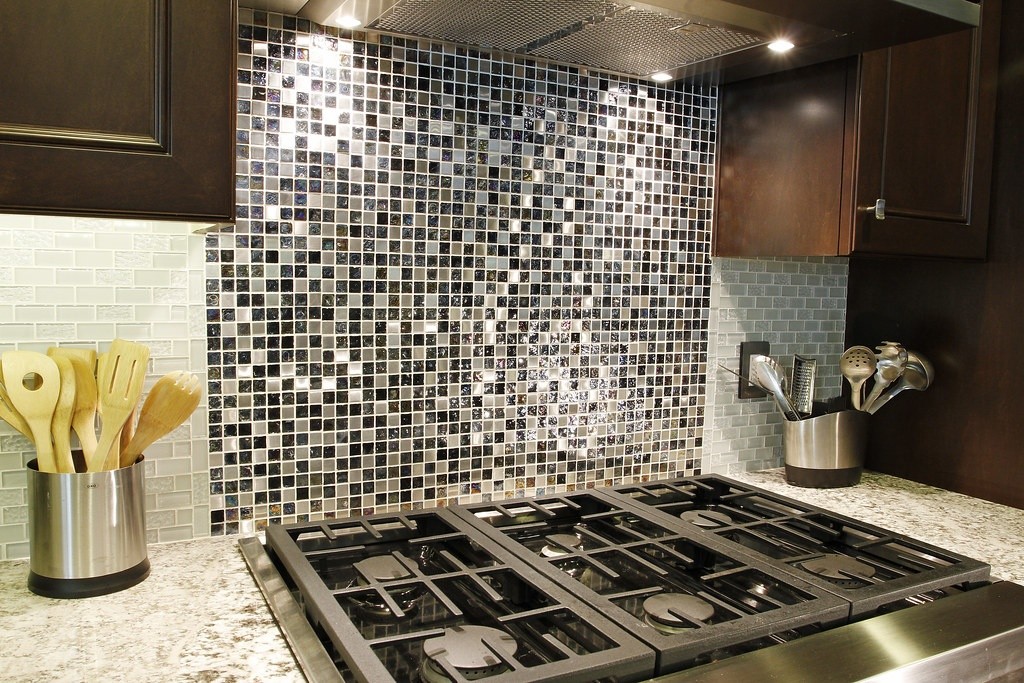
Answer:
[263,507,656,683]
[597,473,992,619]
[446,490,851,667]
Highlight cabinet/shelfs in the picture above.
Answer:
[714,0,1006,263]
[1,1,238,235]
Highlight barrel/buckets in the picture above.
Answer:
[781,401,863,487]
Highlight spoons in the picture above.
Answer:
[840,345,877,410]
[119,371,202,470]
[752,355,802,421]
[868,351,933,413]
[34,355,76,474]
[858,339,909,412]
[65,357,98,469]
[2,351,61,473]
